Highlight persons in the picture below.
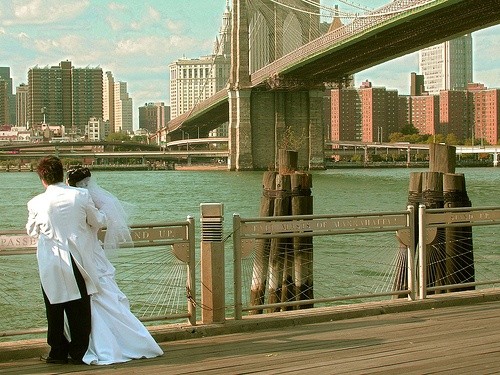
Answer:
[65,165,164,365]
[25,154,108,363]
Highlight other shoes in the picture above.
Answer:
[40,354,67,363]
[68,358,84,364]
